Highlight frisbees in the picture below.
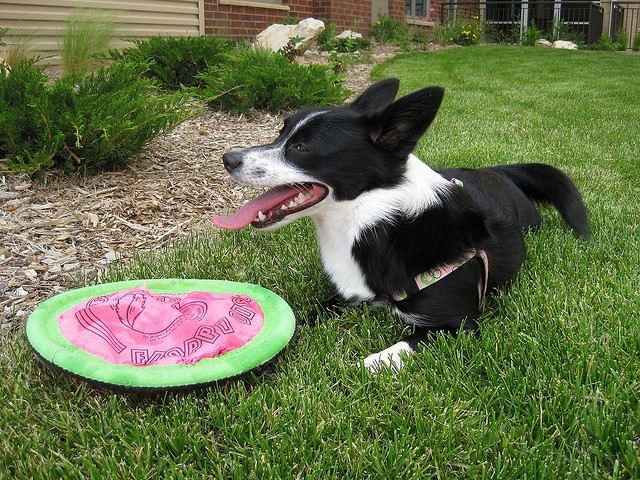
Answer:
[25,279,297,391]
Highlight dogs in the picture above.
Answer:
[213,77,592,379]
